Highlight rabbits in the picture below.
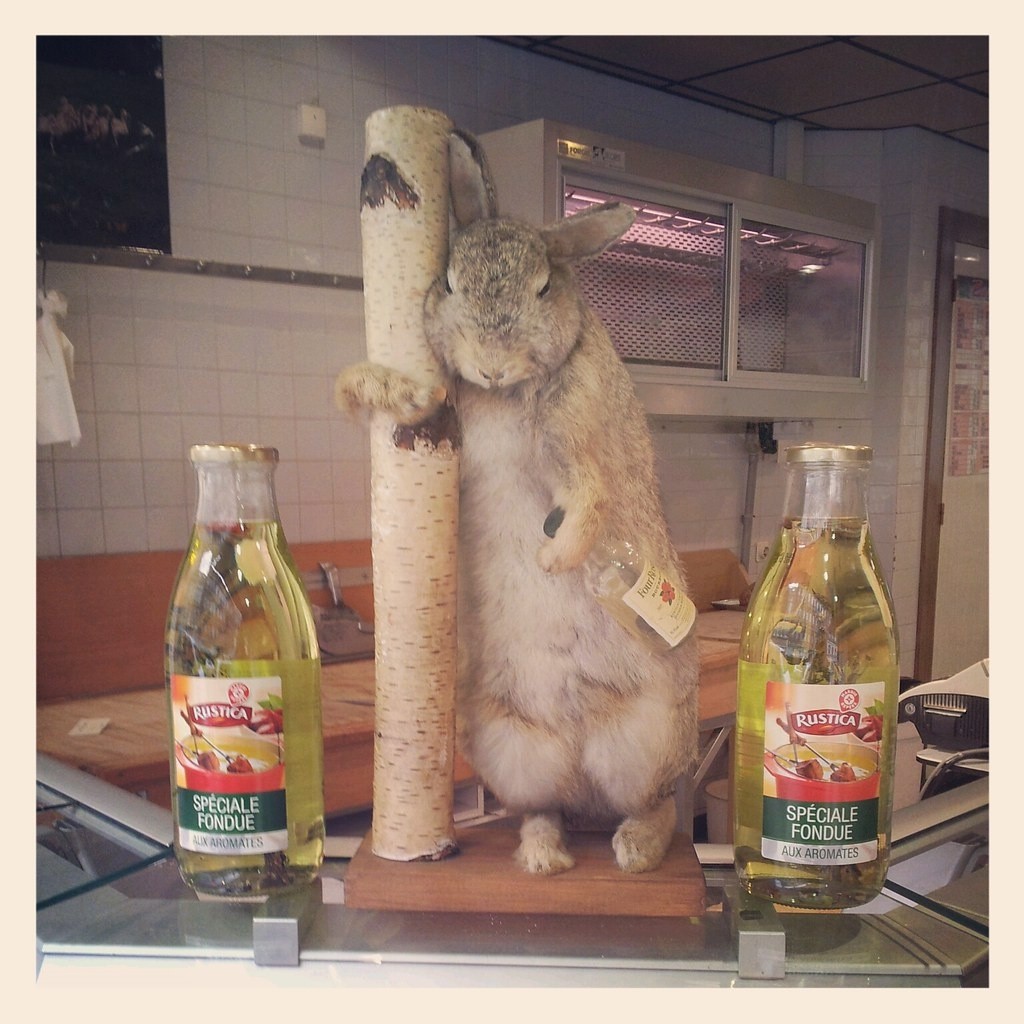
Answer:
[333,128,699,874]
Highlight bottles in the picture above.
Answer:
[165,439,328,896]
[732,440,899,906]
[545,509,701,655]
